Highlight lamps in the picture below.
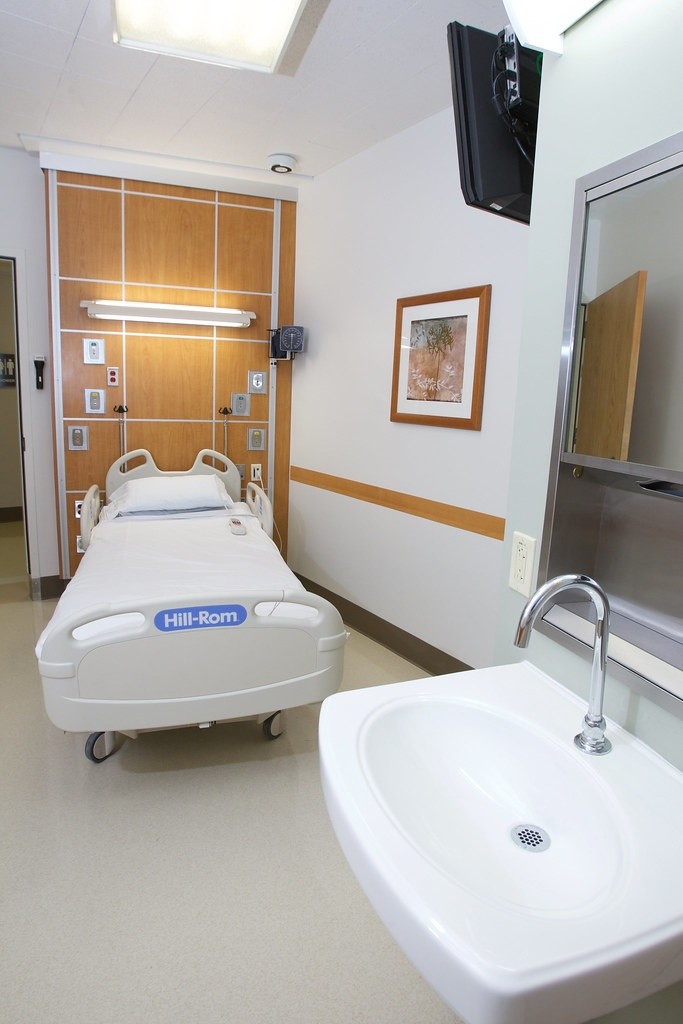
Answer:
[80,299,256,328]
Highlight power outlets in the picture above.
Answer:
[508,531,536,599]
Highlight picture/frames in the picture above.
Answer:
[389,284,494,432]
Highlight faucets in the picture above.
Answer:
[514,573,611,731]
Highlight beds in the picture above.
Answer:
[34,449,350,762]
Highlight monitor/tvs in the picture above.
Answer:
[446,20,543,227]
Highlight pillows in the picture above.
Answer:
[106,474,235,522]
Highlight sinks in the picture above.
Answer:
[319,659,682,1024]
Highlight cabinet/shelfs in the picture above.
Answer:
[529,130,683,723]
